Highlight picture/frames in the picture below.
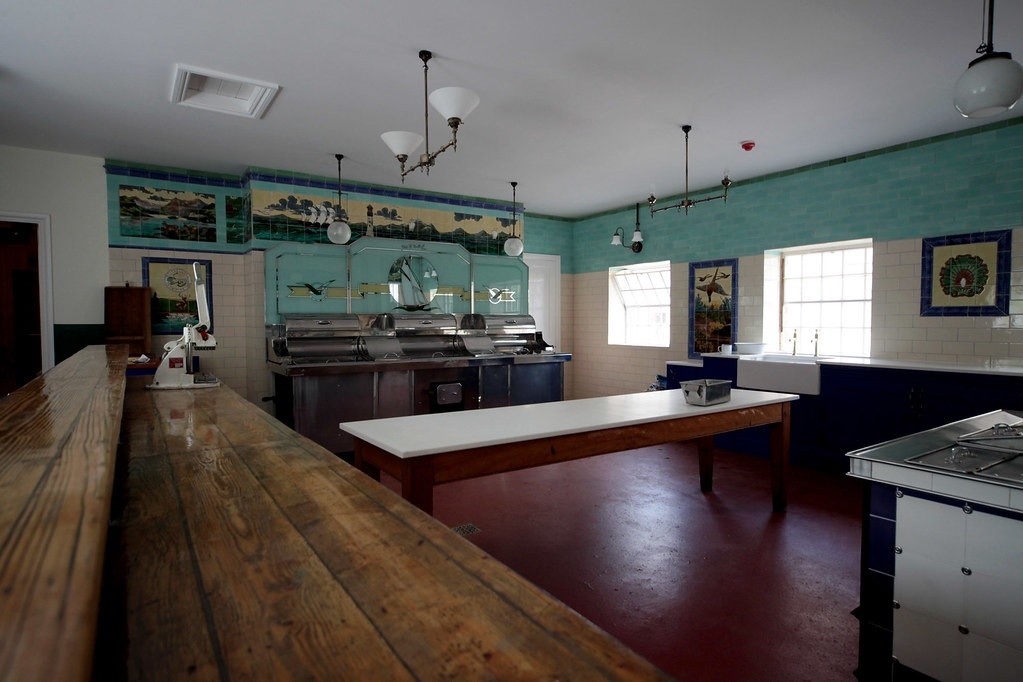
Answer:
[686,258,738,360]
[919,229,1012,317]
[141,256,214,335]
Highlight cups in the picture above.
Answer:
[717,344,732,355]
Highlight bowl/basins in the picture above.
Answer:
[733,342,766,354]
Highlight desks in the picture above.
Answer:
[0,345,687,682]
[344,385,802,520]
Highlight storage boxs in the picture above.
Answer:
[679,378,733,408]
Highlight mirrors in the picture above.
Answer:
[387,256,439,308]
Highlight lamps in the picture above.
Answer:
[380,49,481,185]
[949,0,1023,121]
[504,181,524,257]
[326,154,352,245]
[648,124,732,221]
[610,204,645,254]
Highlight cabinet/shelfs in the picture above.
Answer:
[104,286,152,357]
